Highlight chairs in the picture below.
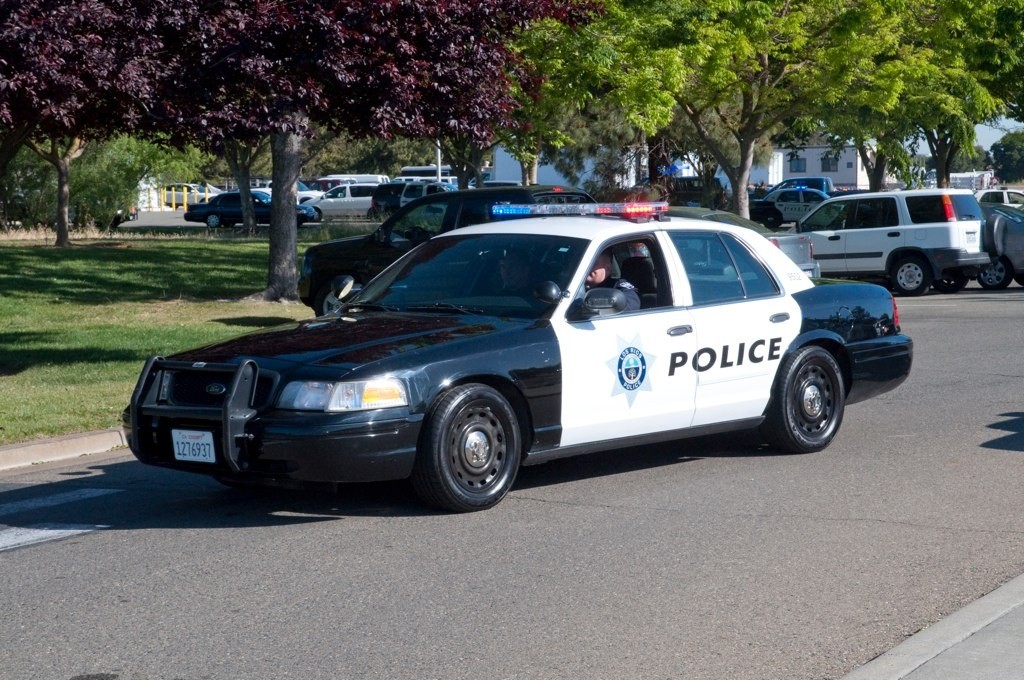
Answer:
[621,255,657,307]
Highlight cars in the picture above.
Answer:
[183,191,319,229]
[249,162,530,225]
[164,182,228,210]
[0,192,138,231]
[974,189,1024,291]
[748,174,873,230]
[120,197,916,516]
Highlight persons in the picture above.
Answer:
[561,246,641,311]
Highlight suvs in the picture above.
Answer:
[295,183,602,316]
[779,184,1008,296]
[625,176,728,213]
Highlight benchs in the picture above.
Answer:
[687,264,759,302]
